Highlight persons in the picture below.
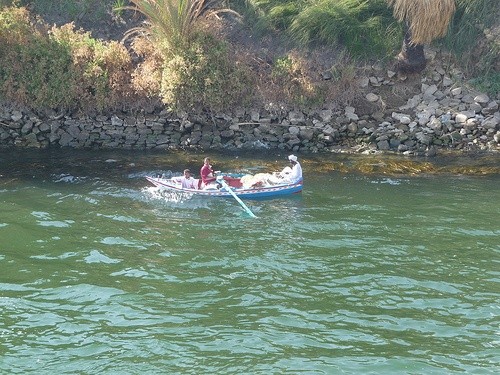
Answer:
[273,154,302,185]
[200,158,229,190]
[170,168,198,191]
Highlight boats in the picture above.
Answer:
[143,175,304,201]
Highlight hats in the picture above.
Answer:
[288,154,299,164]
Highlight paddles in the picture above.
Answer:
[216,176,259,219]
